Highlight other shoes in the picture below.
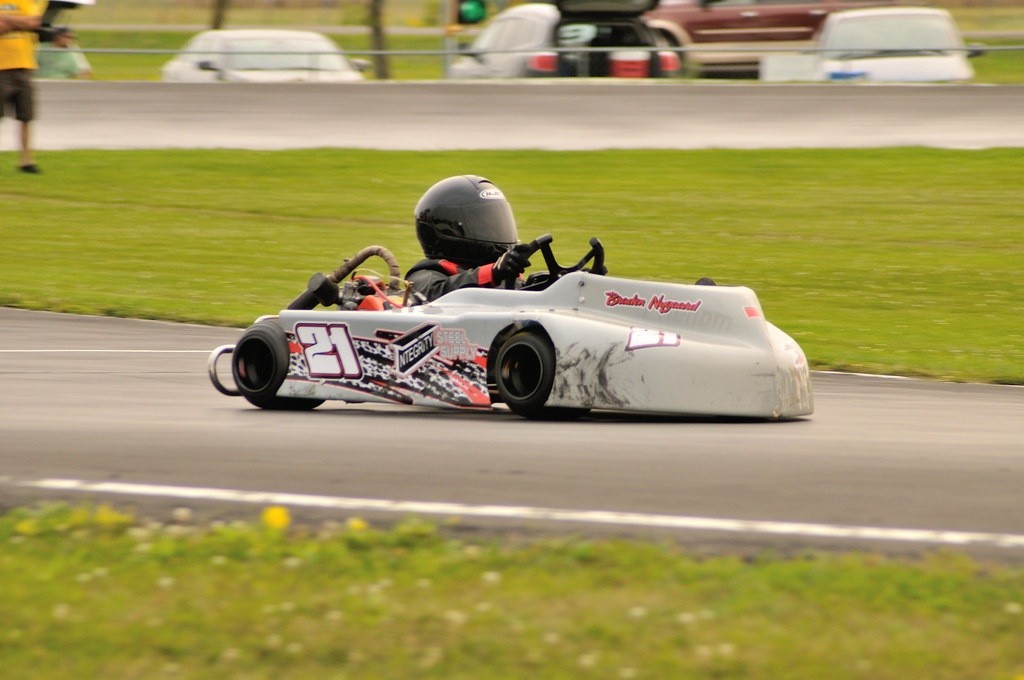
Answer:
[22,164,38,173]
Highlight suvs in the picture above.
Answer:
[640,0,925,70]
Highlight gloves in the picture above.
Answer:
[492,243,531,281]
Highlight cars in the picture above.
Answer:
[803,7,980,82]
[32,0,96,79]
[160,28,372,83]
[449,0,678,79]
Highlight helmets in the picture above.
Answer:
[414,175,518,270]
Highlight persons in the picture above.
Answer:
[1,1,47,176]
[31,29,94,79]
[404,174,609,304]
